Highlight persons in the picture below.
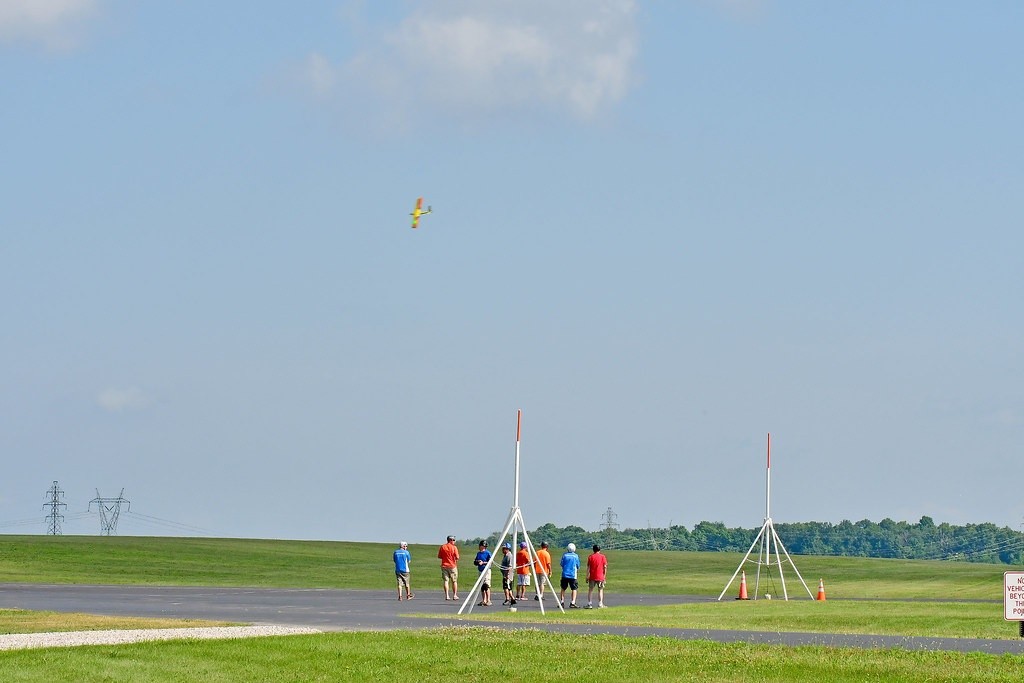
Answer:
[438,535,460,600]
[516,542,531,601]
[557,542,581,609]
[473,540,492,606]
[533,541,552,601]
[392,542,414,601]
[500,542,517,606]
[583,545,608,609]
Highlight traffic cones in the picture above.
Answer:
[814,577,826,602]
[734,568,752,600]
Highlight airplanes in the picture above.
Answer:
[408,196,433,228]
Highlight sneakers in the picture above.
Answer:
[569,602,580,608]
[503,600,510,605]
[598,604,608,609]
[520,596,528,601]
[583,603,593,609]
[515,595,520,601]
[557,602,564,608]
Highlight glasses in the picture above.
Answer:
[451,538,456,542]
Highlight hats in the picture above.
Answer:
[540,541,549,548]
[520,542,527,549]
[447,535,456,541]
[593,545,600,550]
[479,540,488,547]
[502,542,512,550]
[566,543,576,552]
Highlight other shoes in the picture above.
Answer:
[477,601,487,606]
[398,596,403,601]
[446,597,450,600]
[407,595,415,600]
[543,595,545,600]
[483,601,492,606]
[453,596,460,600]
[510,600,517,605]
[534,594,539,601]
